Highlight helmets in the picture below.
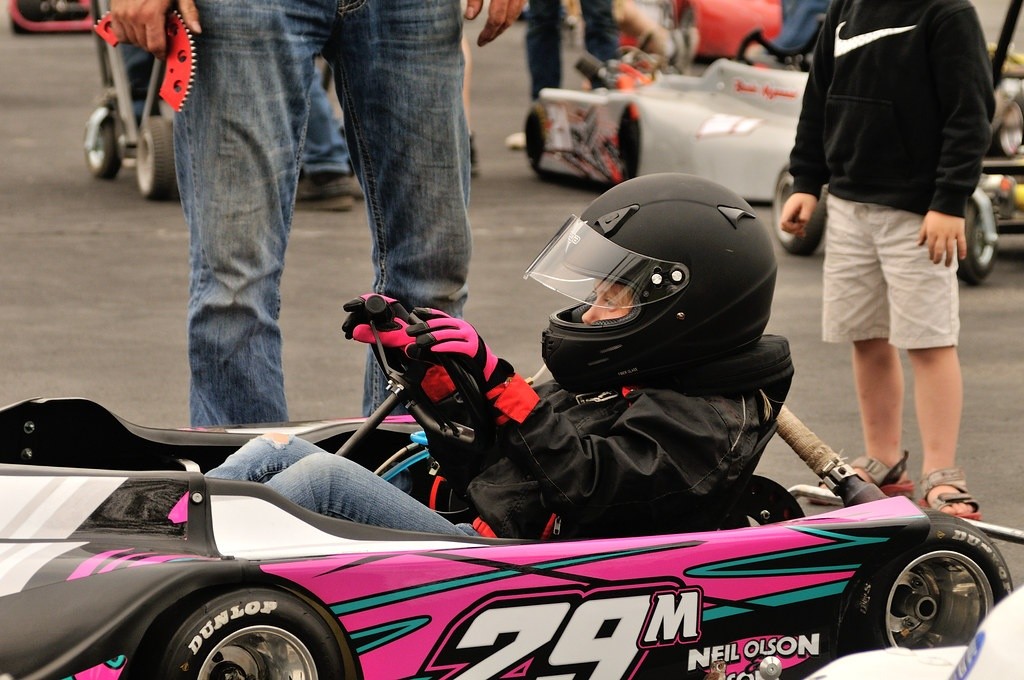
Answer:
[523,171,777,395]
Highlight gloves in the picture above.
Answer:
[342,293,416,352]
[405,304,514,392]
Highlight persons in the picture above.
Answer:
[779,0,995,520]
[108,0,620,431]
[202,172,796,540]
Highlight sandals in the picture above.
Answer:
[919,467,982,521]
[810,450,915,504]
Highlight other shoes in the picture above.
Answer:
[291,180,354,211]
[504,131,527,150]
